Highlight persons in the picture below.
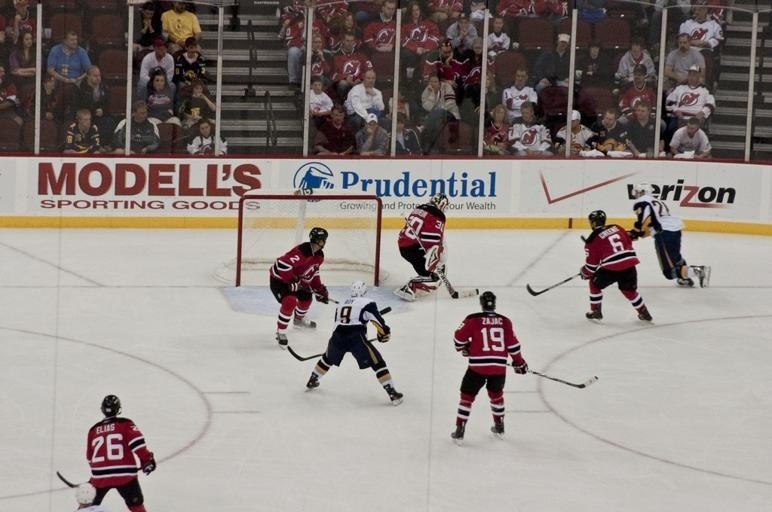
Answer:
[398,193,450,297]
[304,280,404,402]
[450,290,530,441]
[268,227,329,345]
[580,210,654,321]
[272,1,728,158]
[75,482,109,512]
[632,183,706,289]
[1,0,233,157]
[85,394,158,512]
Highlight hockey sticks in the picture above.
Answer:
[581,235,586,242]
[526,273,581,297]
[506,363,598,389]
[56,467,143,488]
[404,217,479,298]
[287,338,377,361]
[300,287,391,316]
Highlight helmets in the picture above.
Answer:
[76,483,95,504]
[480,292,496,309]
[100,394,119,416]
[589,210,606,227]
[632,183,654,201]
[431,193,448,211]
[309,227,328,242]
[351,282,368,297]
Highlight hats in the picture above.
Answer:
[685,64,700,72]
[571,110,580,120]
[154,40,164,47]
[367,113,377,123]
[458,12,469,22]
[634,64,646,74]
[560,34,569,43]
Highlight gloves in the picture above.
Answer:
[289,279,301,292]
[377,332,390,342]
[142,460,155,475]
[315,286,328,304]
[512,361,527,374]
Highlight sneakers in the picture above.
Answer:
[307,376,319,387]
[692,266,704,288]
[400,286,412,295]
[277,334,287,344]
[388,388,403,400]
[293,319,316,328]
[677,278,694,285]
[638,314,651,321]
[491,423,503,433]
[451,427,464,438]
[586,312,602,319]
